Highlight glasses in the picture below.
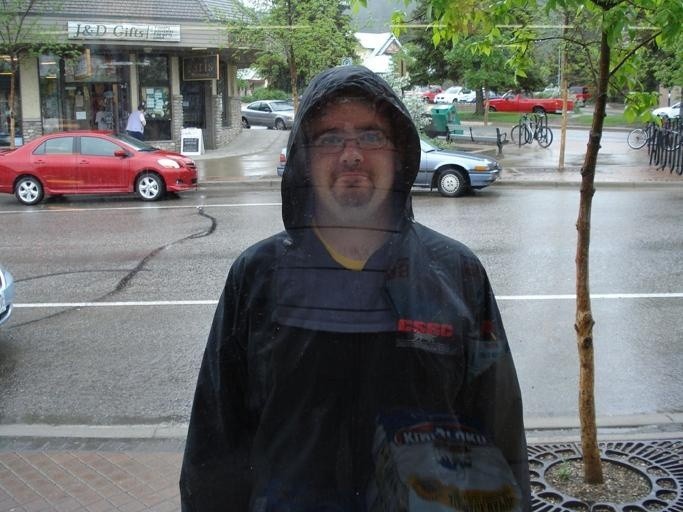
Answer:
[302,132,396,156]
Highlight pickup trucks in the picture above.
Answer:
[483,90,574,114]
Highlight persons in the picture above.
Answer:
[179,64,530,512]
[447,98,458,123]
[126,105,146,141]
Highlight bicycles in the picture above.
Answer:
[627,117,683,175]
[511,114,553,148]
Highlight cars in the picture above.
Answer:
[277,135,500,197]
[241,100,294,130]
[652,101,680,118]
[0,131,197,206]
[415,85,476,103]
[0,266,16,326]
[570,86,592,103]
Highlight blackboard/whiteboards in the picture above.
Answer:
[183,54,219,81]
[180,127,202,156]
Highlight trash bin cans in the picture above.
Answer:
[420,104,464,144]
